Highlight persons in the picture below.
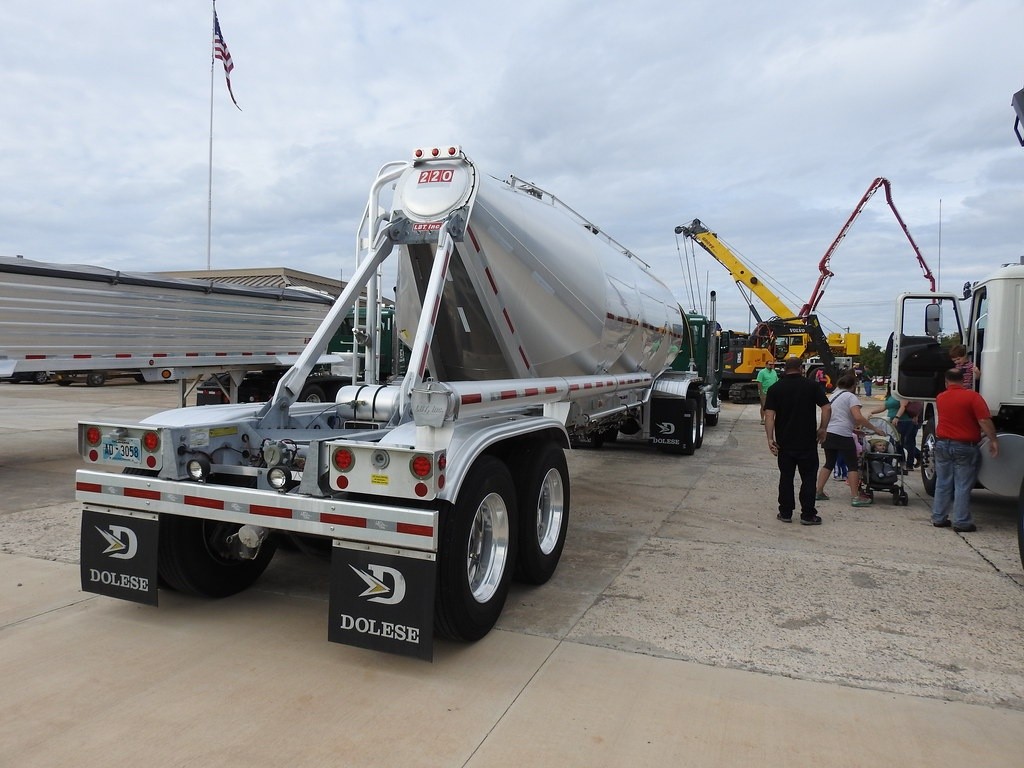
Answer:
[763,357,832,526]
[930,368,999,533]
[756,360,779,425]
[816,362,924,507]
[949,344,981,391]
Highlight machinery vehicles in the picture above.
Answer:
[675,219,860,404]
[798,177,936,318]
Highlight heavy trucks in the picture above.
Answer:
[196,285,411,405]
[76,142,729,664]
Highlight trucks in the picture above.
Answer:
[891,256,1024,569]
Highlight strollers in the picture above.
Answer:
[858,417,909,506]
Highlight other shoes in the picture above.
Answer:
[852,496,871,506]
[776,512,792,522]
[954,525,976,531]
[934,520,950,527]
[898,469,908,475]
[815,492,829,500]
[914,458,922,468]
[906,465,915,471]
[799,515,822,525]
[760,420,765,425]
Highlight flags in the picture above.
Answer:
[215,9,243,113]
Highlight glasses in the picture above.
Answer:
[768,364,773,366]
